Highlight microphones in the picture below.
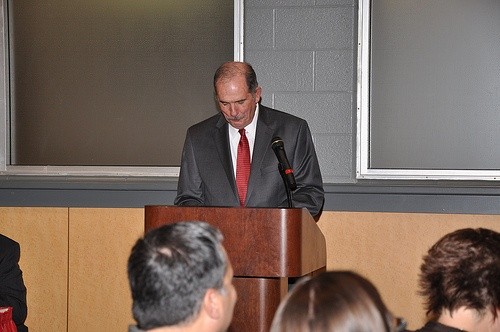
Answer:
[271,136,297,191]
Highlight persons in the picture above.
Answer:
[0,233,29,332]
[174,62,324,218]
[412,228,500,332]
[127,221,238,332]
[269,270,397,332]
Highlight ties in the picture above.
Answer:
[236,129,250,207]
